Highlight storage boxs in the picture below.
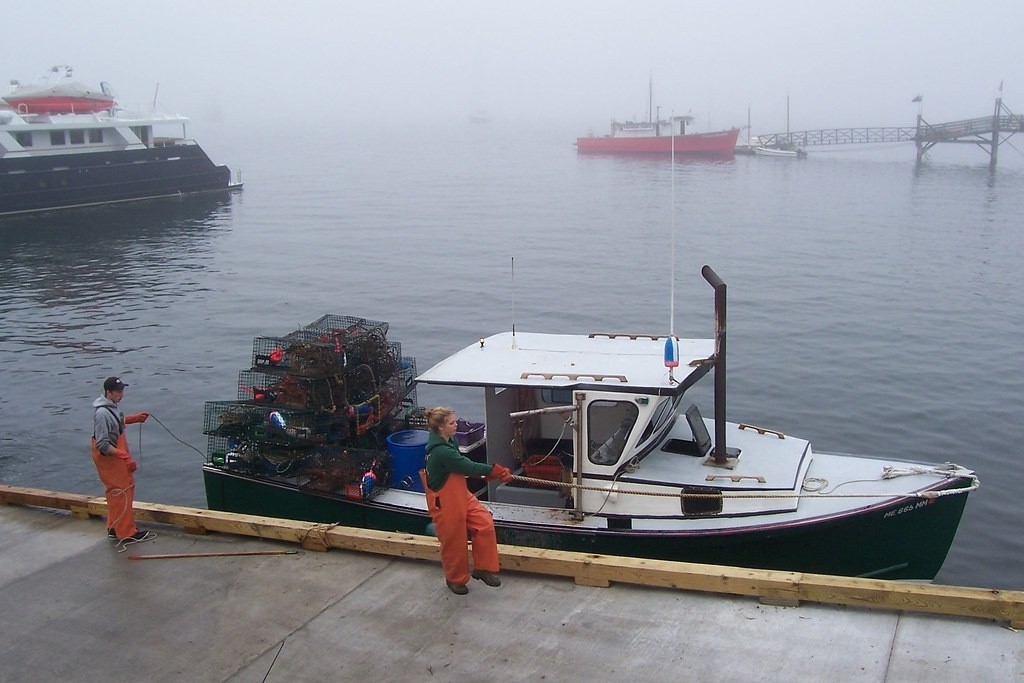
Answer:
[520,455,561,488]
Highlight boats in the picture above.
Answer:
[751,143,806,158]
[200,263,983,584]
[0,60,246,219]
[571,67,746,159]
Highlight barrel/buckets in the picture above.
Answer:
[386,430,430,493]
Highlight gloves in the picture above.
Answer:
[487,463,512,483]
[114,446,137,473]
[124,412,149,424]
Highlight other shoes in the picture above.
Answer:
[107,527,140,538]
[445,578,469,595]
[471,569,502,587]
[118,530,150,544]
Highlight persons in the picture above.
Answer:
[420,407,512,595]
[91,376,149,543]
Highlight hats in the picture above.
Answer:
[103,376,129,390]
